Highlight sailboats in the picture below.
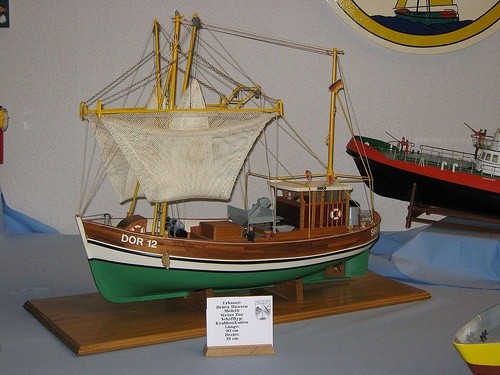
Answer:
[74,10,383,303]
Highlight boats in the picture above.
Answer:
[346,122,500,216]
[452,303,500,375]
